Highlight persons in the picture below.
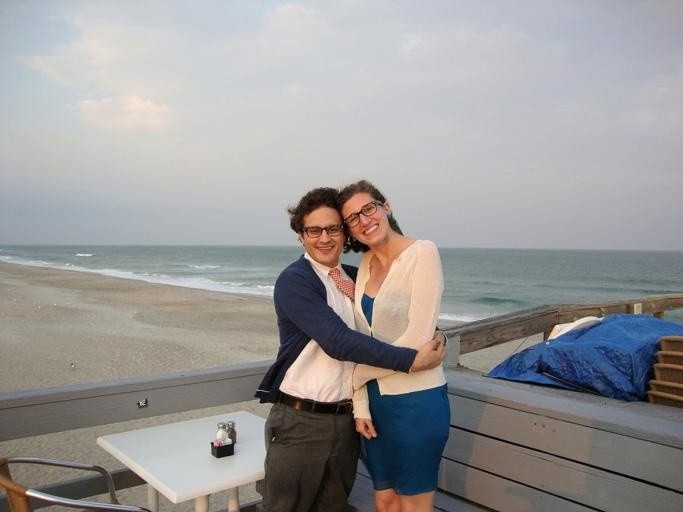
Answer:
[253,188,447,512]
[337,180,451,512]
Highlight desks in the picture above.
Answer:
[96,410,267,512]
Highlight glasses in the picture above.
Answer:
[300,224,344,238]
[342,199,384,228]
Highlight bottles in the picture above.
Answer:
[226,420,236,445]
[214,422,229,446]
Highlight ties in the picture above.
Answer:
[329,269,355,304]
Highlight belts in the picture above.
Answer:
[277,390,353,415]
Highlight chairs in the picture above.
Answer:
[0,457,152,512]
